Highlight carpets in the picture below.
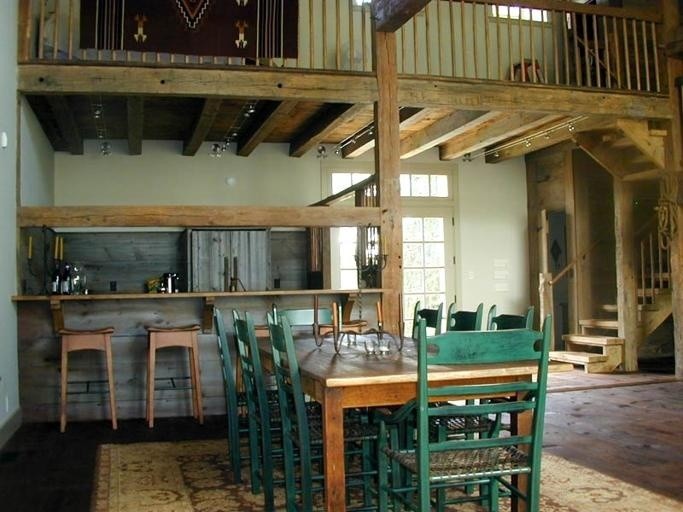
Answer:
[88,432,682,512]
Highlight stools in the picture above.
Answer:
[505,56,546,84]
[57,323,207,430]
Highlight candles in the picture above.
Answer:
[313,291,406,335]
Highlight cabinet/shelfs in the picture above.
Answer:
[177,226,273,292]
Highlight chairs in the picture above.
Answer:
[211,300,535,511]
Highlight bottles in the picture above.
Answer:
[49,263,61,295]
[61,262,72,295]
